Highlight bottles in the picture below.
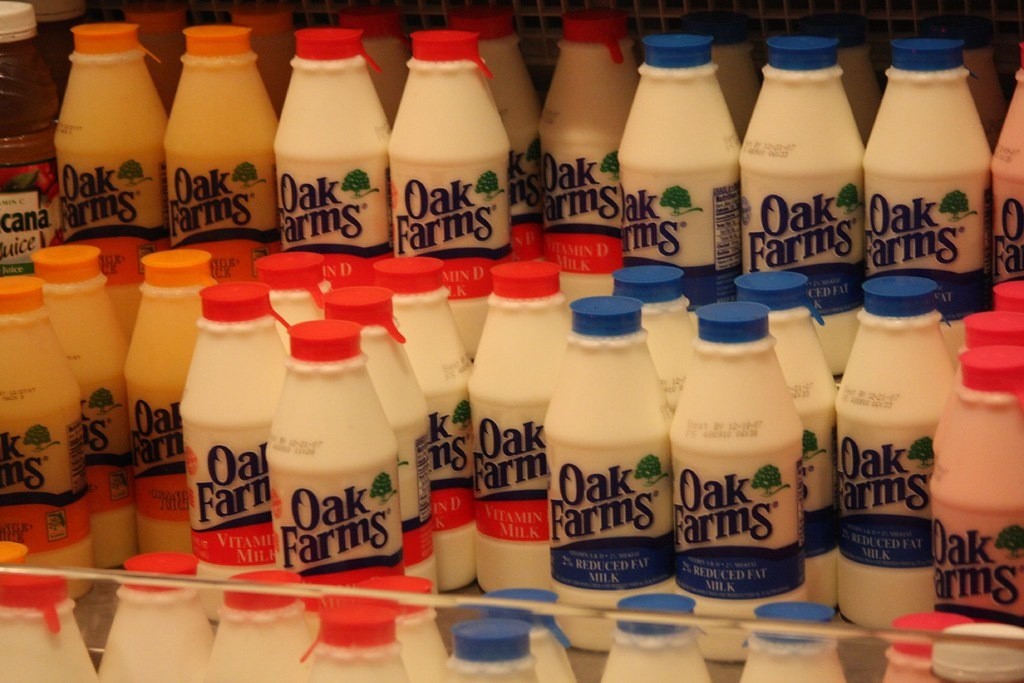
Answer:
[0,0,1024,681]
